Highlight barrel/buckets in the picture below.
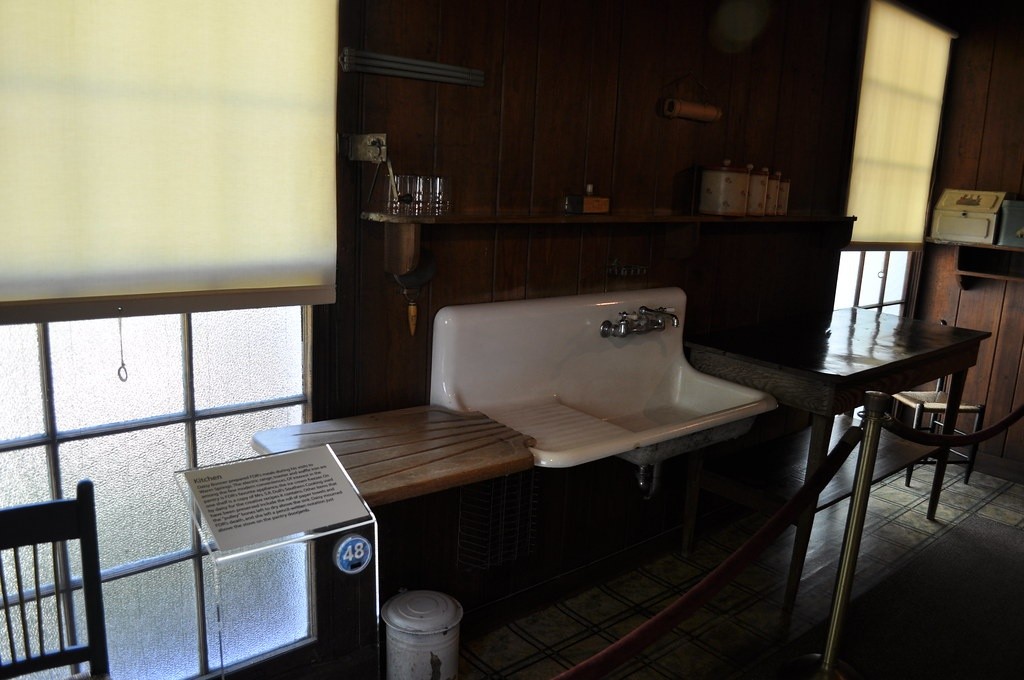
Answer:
[380,589,464,680]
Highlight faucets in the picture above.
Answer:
[657,312,680,328]
[639,305,663,324]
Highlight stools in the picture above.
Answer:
[894,392,983,487]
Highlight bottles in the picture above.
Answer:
[698,158,790,217]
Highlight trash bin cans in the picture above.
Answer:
[380,589,463,680]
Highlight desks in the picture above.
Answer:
[679,306,994,640]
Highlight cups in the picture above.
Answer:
[416,176,448,217]
[388,174,418,217]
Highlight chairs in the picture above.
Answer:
[0,481,112,680]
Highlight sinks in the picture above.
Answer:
[552,365,779,467]
